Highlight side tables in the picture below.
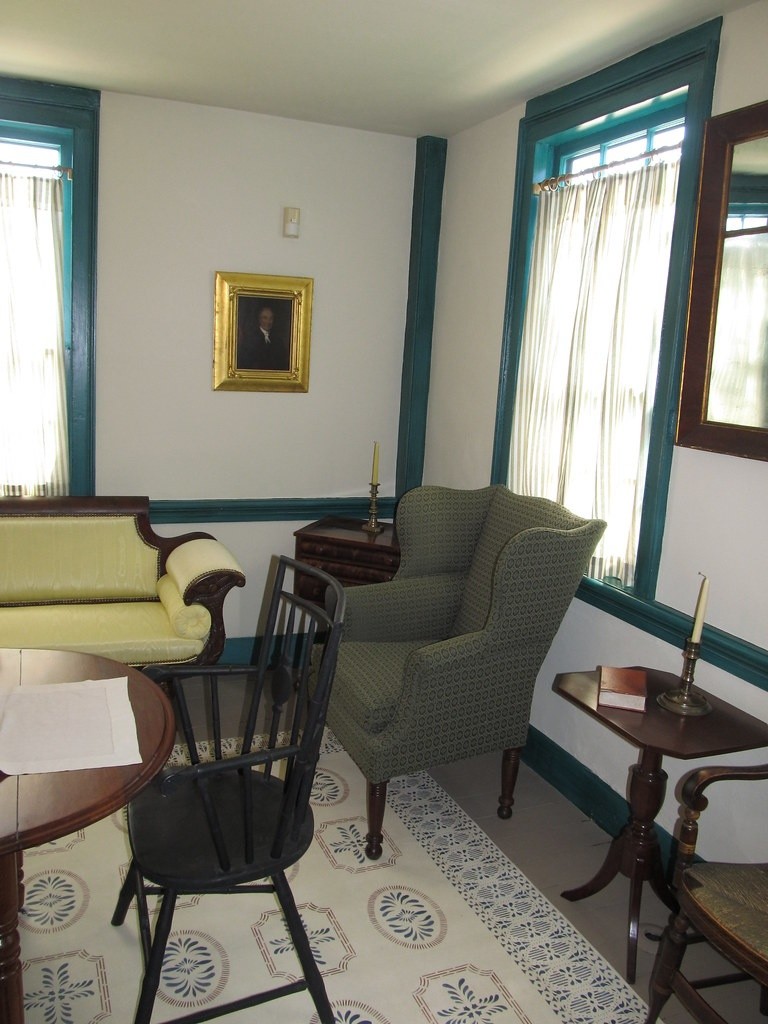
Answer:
[292,515,401,602]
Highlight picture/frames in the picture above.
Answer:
[212,270,314,394]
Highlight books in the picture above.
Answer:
[599,666,648,712]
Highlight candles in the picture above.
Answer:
[371,442,380,485]
[692,571,709,641]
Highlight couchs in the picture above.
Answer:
[0,494,244,730]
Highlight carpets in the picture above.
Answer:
[17,728,666,1024]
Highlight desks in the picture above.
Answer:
[0,650,179,1024]
[551,666,768,983]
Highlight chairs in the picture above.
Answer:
[113,555,348,1024]
[647,762,768,1024]
[311,483,609,859]
